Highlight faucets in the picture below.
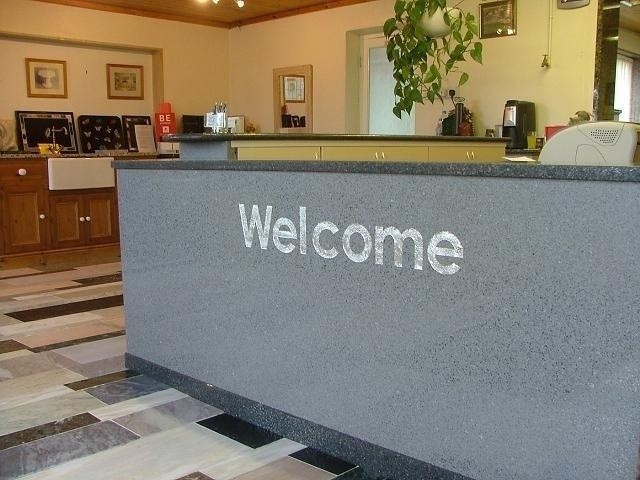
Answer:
[48,126,68,154]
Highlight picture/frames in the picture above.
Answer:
[105,62,146,100]
[282,75,305,103]
[477,1,518,40]
[25,58,71,100]
[15,110,79,155]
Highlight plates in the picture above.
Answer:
[523,149,540,151]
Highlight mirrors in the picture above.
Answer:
[273,64,315,133]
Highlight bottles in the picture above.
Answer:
[436,111,448,135]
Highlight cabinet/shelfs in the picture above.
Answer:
[0,158,116,255]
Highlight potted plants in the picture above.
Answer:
[380,0,485,124]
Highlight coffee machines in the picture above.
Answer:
[502,99,536,149]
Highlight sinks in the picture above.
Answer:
[48,156,116,192]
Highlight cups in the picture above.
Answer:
[527,131,537,149]
